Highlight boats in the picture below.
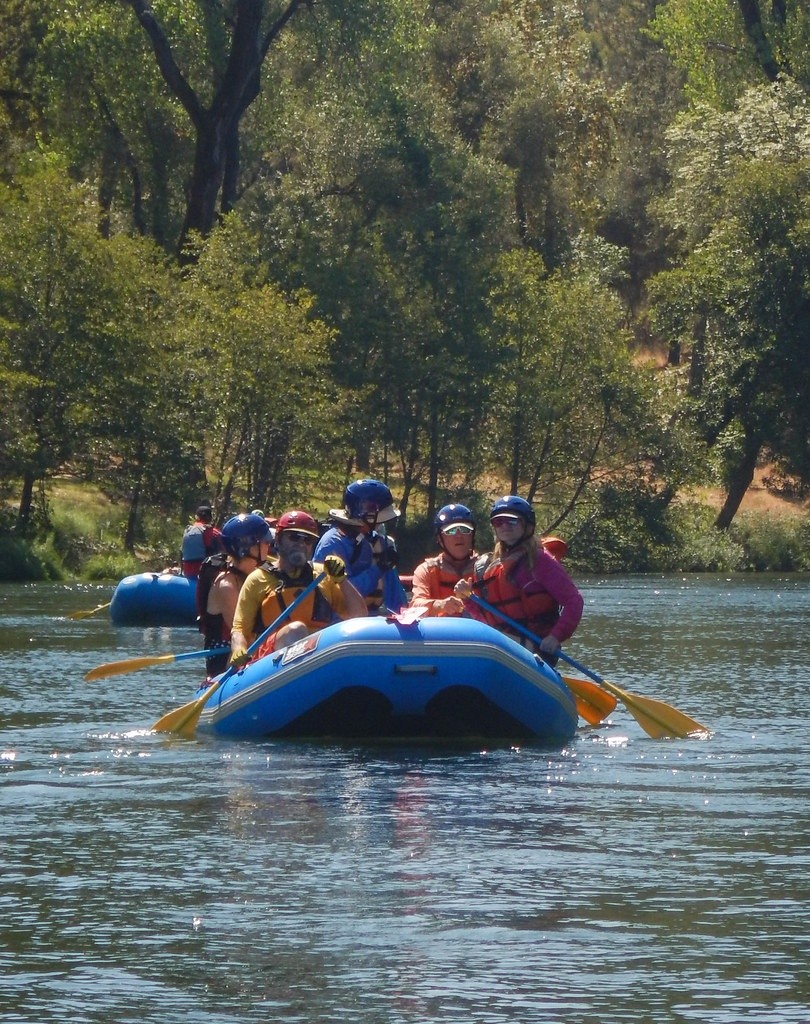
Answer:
[110,568,198,627]
[193,609,578,750]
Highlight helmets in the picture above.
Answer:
[220,513,270,560]
[344,479,393,519]
[276,511,321,538]
[432,503,474,528]
[490,495,536,526]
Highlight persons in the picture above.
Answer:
[411,504,480,617]
[312,480,407,628]
[182,506,368,677]
[453,495,583,667]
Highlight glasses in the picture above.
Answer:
[441,526,472,535]
[284,532,316,545]
[492,517,520,526]
[260,530,273,544]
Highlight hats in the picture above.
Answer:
[440,522,474,531]
[251,509,265,518]
[490,511,522,520]
[329,503,400,526]
[196,506,212,516]
[282,528,320,538]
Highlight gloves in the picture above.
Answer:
[324,555,347,583]
[230,648,249,666]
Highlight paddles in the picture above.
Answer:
[560,674,618,725]
[148,567,329,737]
[82,643,231,682]
[68,595,111,621]
[467,590,707,738]
